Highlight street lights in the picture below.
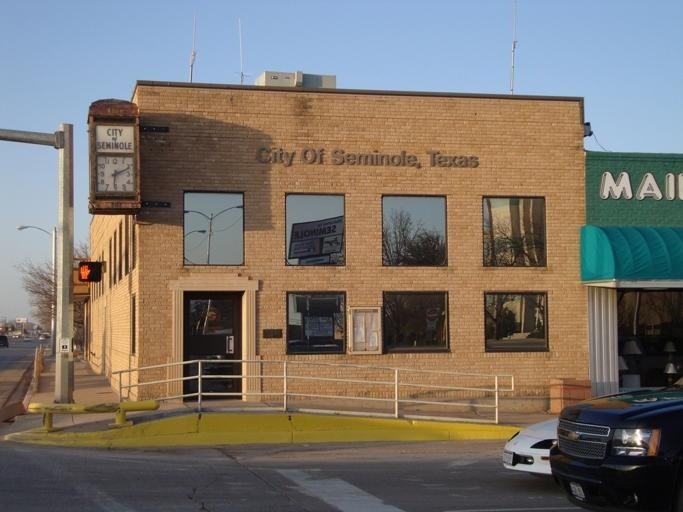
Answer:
[18,226,57,358]
[184,204,244,265]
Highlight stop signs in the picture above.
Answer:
[425,307,441,327]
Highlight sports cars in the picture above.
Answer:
[502,416,560,478]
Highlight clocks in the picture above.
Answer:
[86,98,142,215]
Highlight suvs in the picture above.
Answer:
[549,370,683,511]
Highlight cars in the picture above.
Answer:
[12,334,45,343]
[0,335,9,349]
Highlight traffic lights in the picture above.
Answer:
[78,262,103,283]
